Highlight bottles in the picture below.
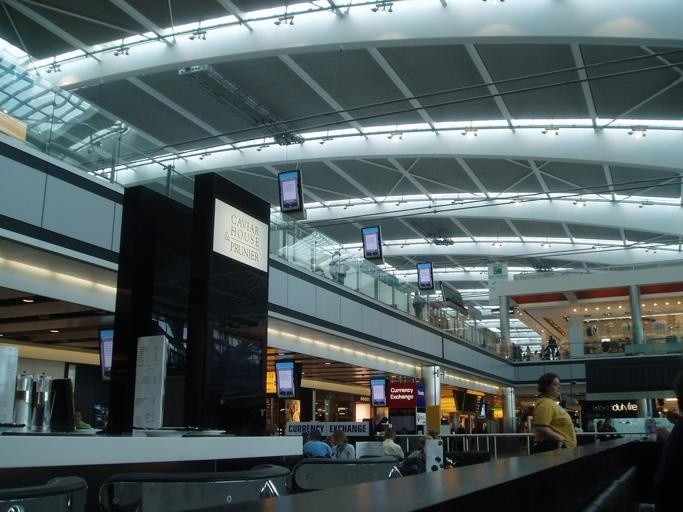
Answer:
[29,372,53,431]
[12,370,33,428]
[644,412,656,440]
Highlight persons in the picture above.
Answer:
[327,430,355,460]
[525,344,530,353]
[411,289,425,321]
[299,427,333,460]
[67,407,94,433]
[540,344,546,360]
[649,367,682,512]
[543,335,556,352]
[530,373,576,452]
[643,419,669,441]
[594,418,624,444]
[406,435,434,458]
[331,249,350,284]
[382,428,405,459]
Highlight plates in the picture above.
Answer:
[138,428,191,437]
[74,428,103,434]
[191,430,225,435]
